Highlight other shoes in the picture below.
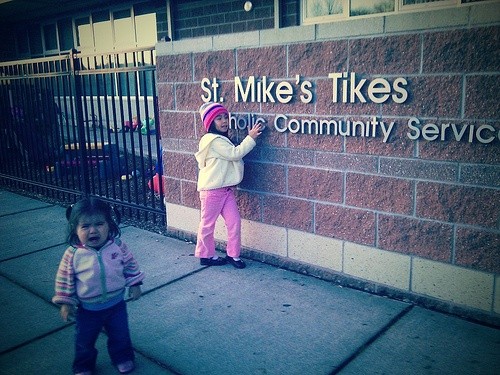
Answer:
[200,256,226,266]
[75,371,93,375]
[225,255,246,268]
[117,360,135,373]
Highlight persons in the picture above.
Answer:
[192,101,263,269]
[50,196,146,375]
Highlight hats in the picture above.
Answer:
[199,102,226,132]
